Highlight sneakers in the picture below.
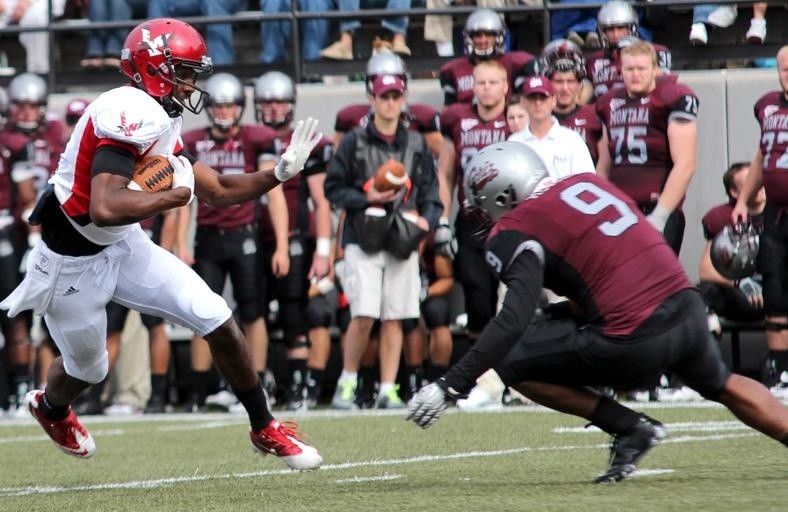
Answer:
[244,418,323,472]
[435,39,456,61]
[593,412,667,487]
[370,382,405,411]
[708,7,741,27]
[329,376,357,410]
[389,37,413,58]
[203,383,242,411]
[102,399,147,421]
[689,21,710,47]
[229,391,276,420]
[142,390,167,416]
[287,384,310,414]
[24,388,98,461]
[744,18,769,45]
[320,41,358,63]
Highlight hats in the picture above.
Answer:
[65,97,92,126]
[521,73,558,101]
[369,74,410,97]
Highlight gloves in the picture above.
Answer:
[402,378,461,430]
[731,271,763,307]
[430,219,461,260]
[271,116,325,182]
[162,154,199,208]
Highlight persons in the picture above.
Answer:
[402,139,788,483]
[25,16,326,470]
[0,0,788,409]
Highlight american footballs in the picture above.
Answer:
[373,163,407,191]
[128,156,177,194]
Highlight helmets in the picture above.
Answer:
[707,220,764,282]
[253,69,298,128]
[460,8,508,59]
[595,0,640,47]
[539,38,586,80]
[115,15,215,120]
[460,139,559,244]
[202,71,246,132]
[8,71,49,133]
[363,49,411,91]
